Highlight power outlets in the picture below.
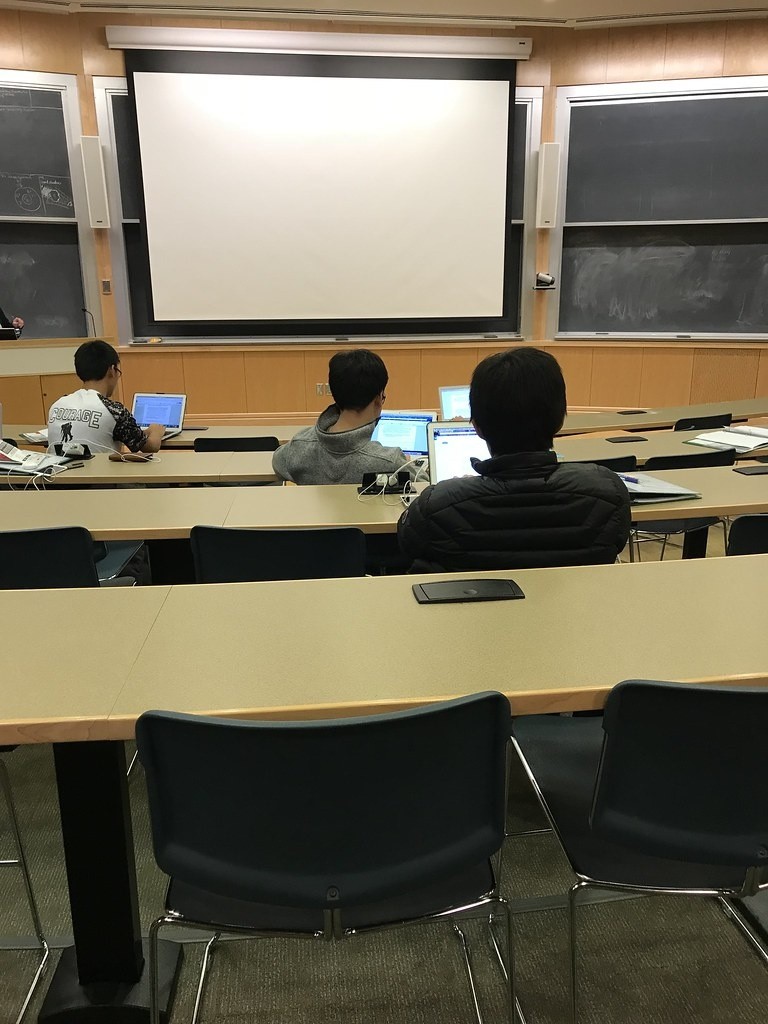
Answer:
[54,444,95,460]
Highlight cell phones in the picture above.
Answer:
[108,451,153,462]
[400,494,419,506]
[33,465,68,476]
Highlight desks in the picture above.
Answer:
[0,422,768,1024]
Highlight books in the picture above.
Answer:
[683,425,768,453]
[618,474,701,506]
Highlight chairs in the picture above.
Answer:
[629,449,737,563]
[134,691,517,1024]
[0,526,149,590]
[487,679,768,1024]
[189,524,366,584]
[673,413,732,431]
[557,455,636,563]
[194,436,286,486]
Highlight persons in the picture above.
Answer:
[0,308,24,338]
[272,349,429,482]
[47,339,165,454]
[395,347,631,571]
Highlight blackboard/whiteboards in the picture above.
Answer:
[543,73,768,341]
[92,73,542,345]
[0,69,107,348]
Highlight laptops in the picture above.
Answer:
[371,385,493,486]
[130,393,188,440]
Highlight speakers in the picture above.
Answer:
[81,135,111,228]
[536,142,560,229]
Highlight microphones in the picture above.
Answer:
[81,307,97,338]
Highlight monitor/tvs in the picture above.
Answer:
[0,328,18,340]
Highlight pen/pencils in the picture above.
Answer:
[618,474,639,483]
[66,463,84,470]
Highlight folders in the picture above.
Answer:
[616,474,702,506]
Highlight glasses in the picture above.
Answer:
[377,392,385,405]
[110,362,122,377]
